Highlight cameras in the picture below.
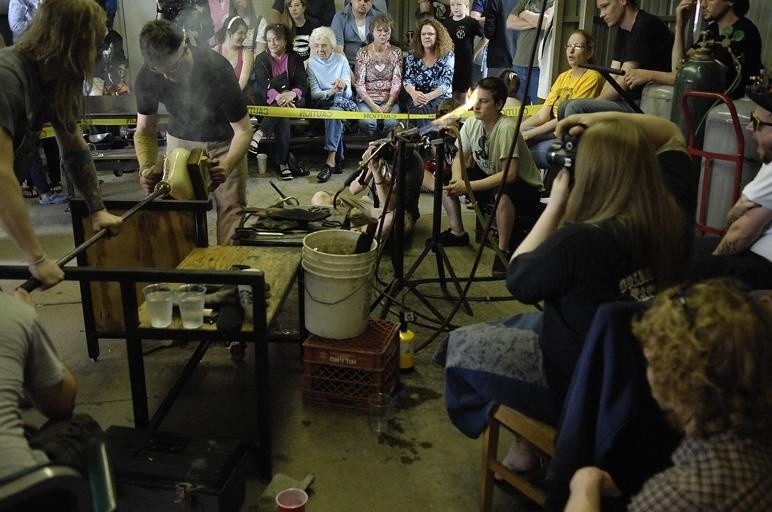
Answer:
[544,135,575,181]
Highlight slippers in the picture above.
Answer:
[48,179,62,193]
[21,184,41,198]
[490,459,549,490]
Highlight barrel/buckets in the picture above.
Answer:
[300,228,379,340]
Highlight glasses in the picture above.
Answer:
[749,111,772,132]
[564,43,587,50]
[477,135,490,161]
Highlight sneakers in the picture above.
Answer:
[425,227,470,248]
[279,167,293,180]
[247,139,260,155]
[316,157,346,183]
[491,248,510,275]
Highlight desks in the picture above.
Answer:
[42,95,171,204]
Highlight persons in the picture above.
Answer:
[0,0,125,282]
[438,109,708,427]
[151,2,763,183]
[132,20,254,249]
[420,78,541,278]
[0,286,112,486]
[695,75,772,296]
[312,123,426,253]
[565,271,772,511]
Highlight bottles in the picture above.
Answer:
[395,310,417,374]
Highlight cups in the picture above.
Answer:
[142,283,175,329]
[275,487,309,512]
[256,153,268,174]
[367,392,396,434]
[175,284,208,331]
[237,268,262,319]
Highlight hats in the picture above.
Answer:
[745,77,772,113]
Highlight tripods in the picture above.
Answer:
[391,159,475,319]
[370,194,454,332]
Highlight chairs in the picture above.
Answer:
[480,296,686,512]
